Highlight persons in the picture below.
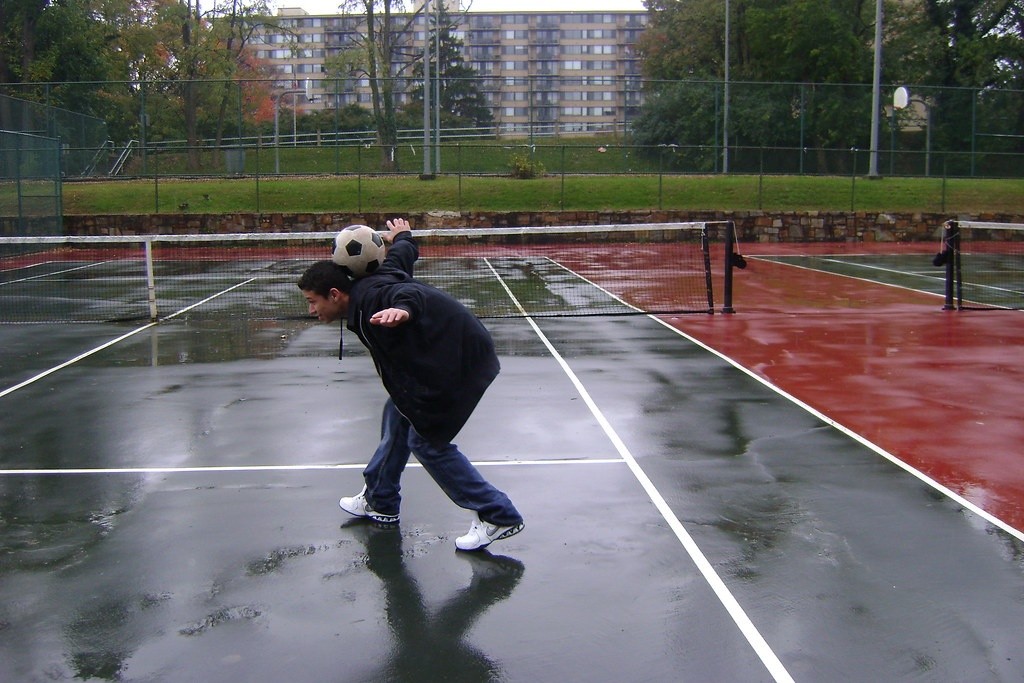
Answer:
[298,218,525,552]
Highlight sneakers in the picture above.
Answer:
[454,549,525,582]
[340,522,400,545]
[339,482,401,522]
[455,515,525,550]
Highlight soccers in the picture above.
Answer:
[330,223,386,274]
